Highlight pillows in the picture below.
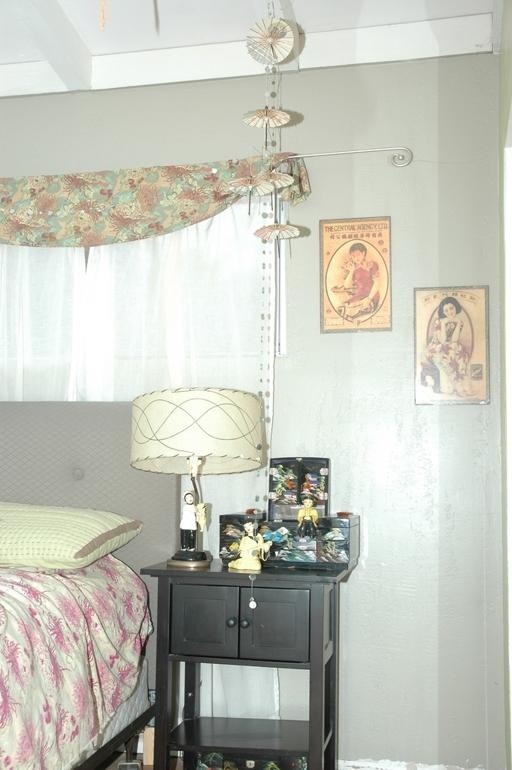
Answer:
[0,500,144,570]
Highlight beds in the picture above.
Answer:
[0,402,181,770]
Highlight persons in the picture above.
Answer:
[428,298,473,394]
[229,520,274,573]
[336,240,380,314]
[178,491,199,553]
[295,496,320,528]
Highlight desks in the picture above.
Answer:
[139,559,355,770]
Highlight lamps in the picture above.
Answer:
[130,388,268,567]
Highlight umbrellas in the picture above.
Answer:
[258,172,294,212]
[224,175,275,217]
[244,17,295,66]
[239,106,290,153]
[254,220,302,259]
